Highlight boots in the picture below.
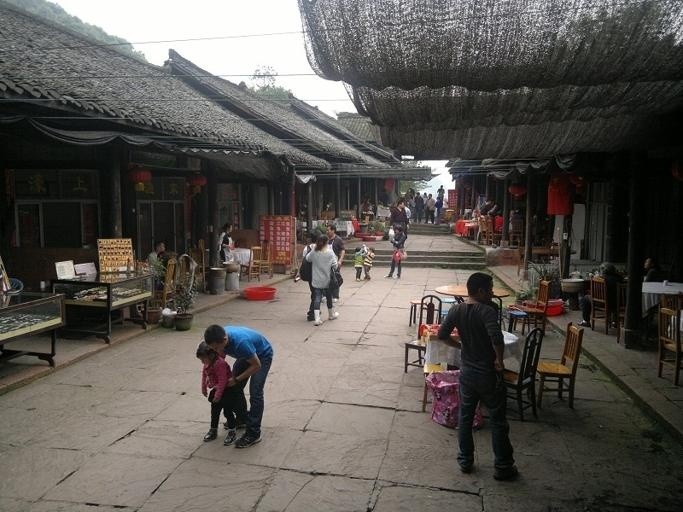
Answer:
[328,308,339,320]
[314,309,323,325]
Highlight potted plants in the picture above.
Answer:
[141,256,167,325]
[168,274,197,331]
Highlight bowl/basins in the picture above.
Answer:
[560,278,584,293]
[544,300,564,315]
[355,233,370,237]
[244,286,276,301]
[361,235,376,241]
[375,232,383,236]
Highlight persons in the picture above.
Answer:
[196,341,237,446]
[205,324,273,449]
[643,257,663,282]
[217,222,235,262]
[438,272,519,482]
[146,239,168,287]
[297,185,444,327]
[476,198,555,245]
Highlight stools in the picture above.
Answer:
[508,311,530,330]
[441,298,457,318]
[422,362,444,412]
[409,300,428,326]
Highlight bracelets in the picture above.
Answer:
[233,376,240,385]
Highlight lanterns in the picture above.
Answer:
[547,177,571,215]
[129,168,151,192]
[384,178,394,193]
[508,184,528,200]
[189,174,207,194]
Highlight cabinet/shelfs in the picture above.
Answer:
[51,270,154,343]
[0,292,64,369]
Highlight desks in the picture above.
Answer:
[435,285,511,328]
[424,330,522,374]
[205,247,253,280]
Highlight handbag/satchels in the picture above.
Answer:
[330,268,343,289]
[393,248,407,264]
[299,246,312,281]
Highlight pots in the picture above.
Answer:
[222,260,241,273]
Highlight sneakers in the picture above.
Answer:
[204,429,217,441]
[224,419,261,447]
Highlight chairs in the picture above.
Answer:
[3,277,24,292]
[505,328,542,419]
[480,216,523,247]
[514,278,550,335]
[589,274,683,391]
[538,322,584,410]
[405,295,443,373]
[240,246,262,282]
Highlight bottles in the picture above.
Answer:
[663,279,667,286]
